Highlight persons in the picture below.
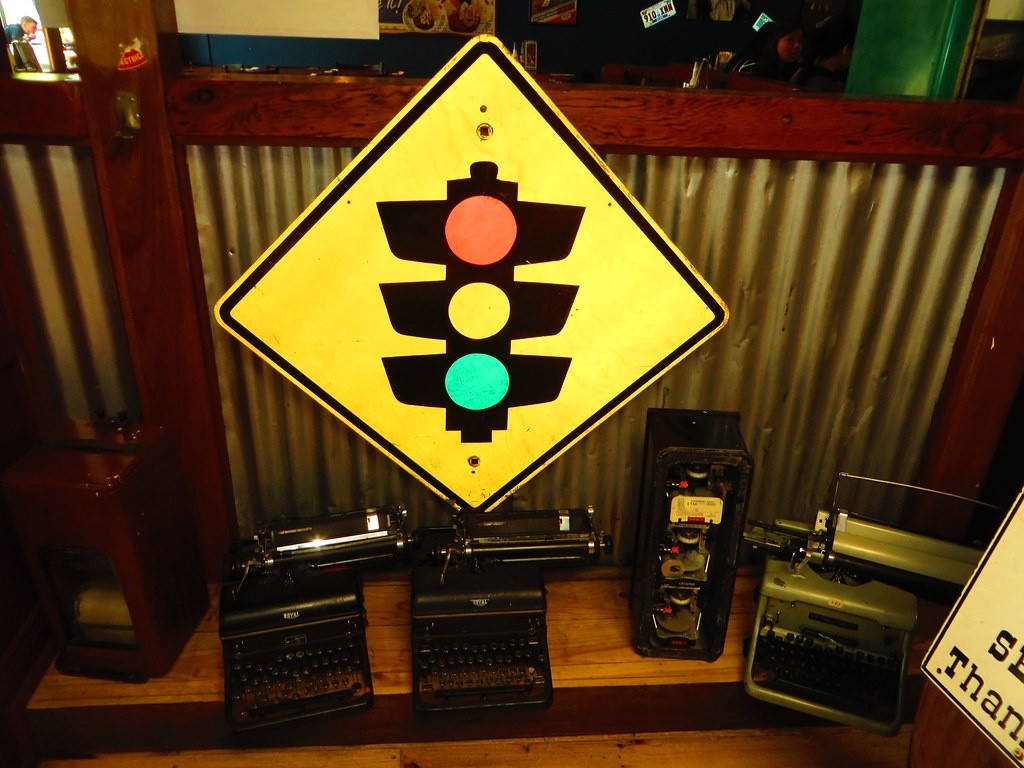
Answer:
[3,18,38,44]
[727,24,855,93]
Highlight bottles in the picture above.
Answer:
[520,42,525,64]
[512,42,518,59]
[690,61,699,88]
